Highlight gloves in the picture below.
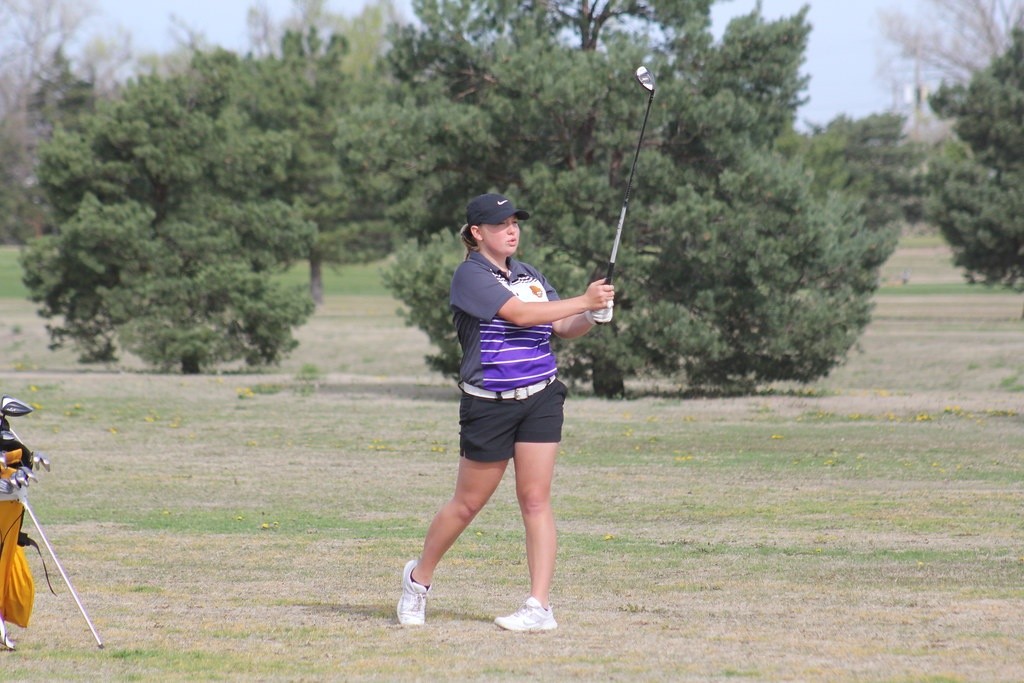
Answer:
[584,300,615,326]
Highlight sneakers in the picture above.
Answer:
[492,597,558,631]
[396,560,432,626]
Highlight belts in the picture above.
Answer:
[462,374,556,400]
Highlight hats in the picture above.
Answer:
[466,193,530,225]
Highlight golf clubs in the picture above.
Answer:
[0,396,51,493]
[606,64,656,285]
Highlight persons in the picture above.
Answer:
[396,194,615,633]
